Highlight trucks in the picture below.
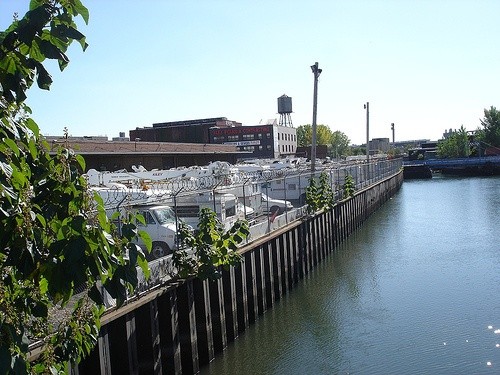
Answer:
[80,153,388,268]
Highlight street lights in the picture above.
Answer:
[364,102,369,179]
[309,61,322,186]
[391,123,394,159]
